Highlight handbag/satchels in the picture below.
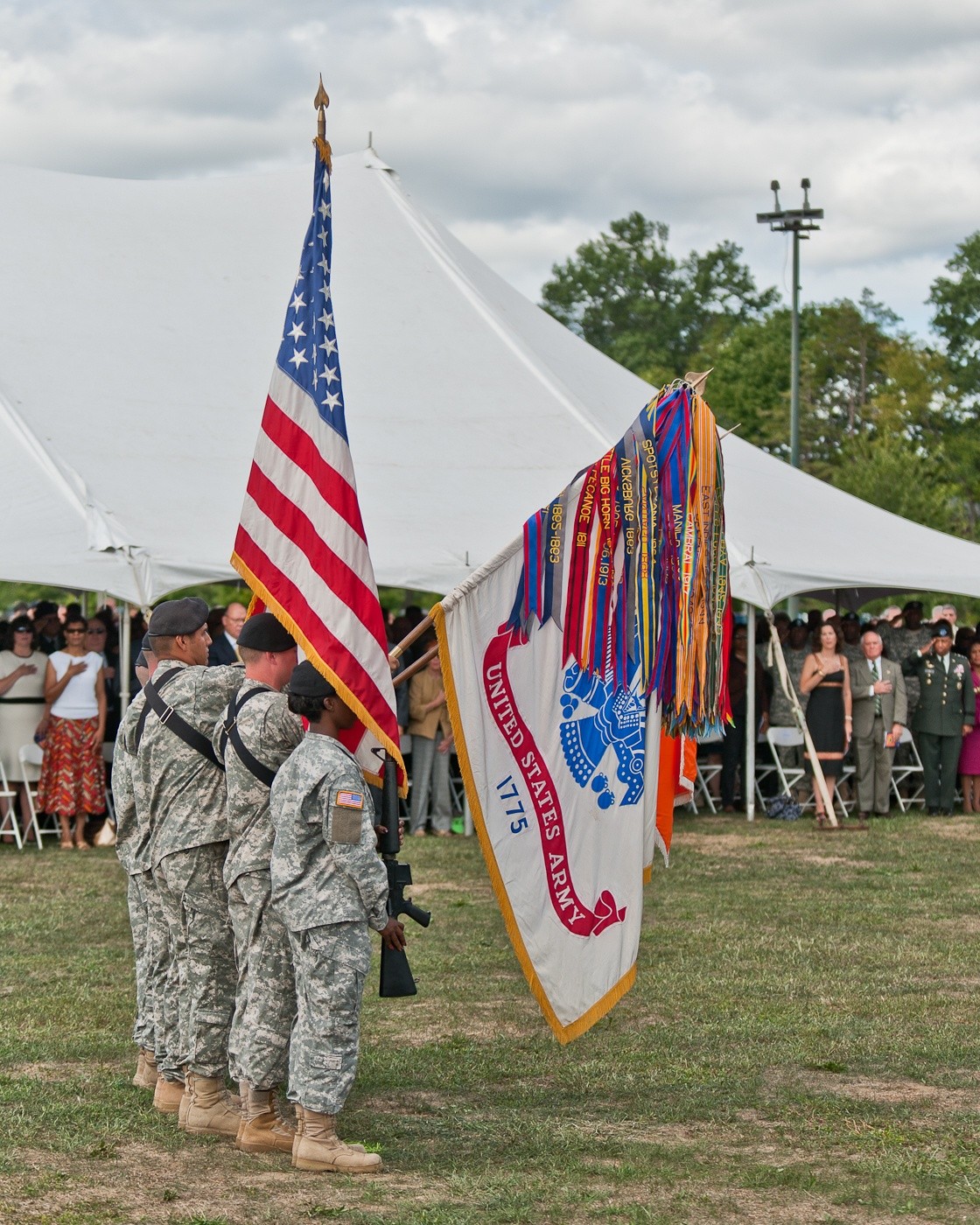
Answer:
[92,817,117,847]
[768,794,802,822]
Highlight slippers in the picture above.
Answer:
[60,840,91,851]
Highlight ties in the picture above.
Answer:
[940,656,946,672]
[870,660,881,715]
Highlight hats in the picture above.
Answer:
[288,660,338,697]
[142,635,151,650]
[134,650,147,666]
[790,619,806,629]
[932,619,954,638]
[842,612,859,621]
[237,613,297,653]
[33,600,58,617]
[147,596,208,636]
[903,601,923,615]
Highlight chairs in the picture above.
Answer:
[19,744,77,850]
[753,727,856,818]
[686,726,741,814]
[400,732,464,823]
[0,761,23,849]
[101,743,116,823]
[889,726,974,813]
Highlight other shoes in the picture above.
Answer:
[414,828,425,837]
[432,828,453,837]
[725,803,980,818]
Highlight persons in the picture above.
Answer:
[0,600,980,850]
[109,599,407,1171]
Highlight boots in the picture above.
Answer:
[132,1047,383,1172]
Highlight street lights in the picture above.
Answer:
[756,177,825,620]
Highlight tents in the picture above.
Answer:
[0,132,980,830]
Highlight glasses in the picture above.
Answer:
[226,615,246,623]
[734,633,747,640]
[65,627,86,634]
[13,627,35,633]
[87,628,105,635]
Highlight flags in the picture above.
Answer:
[228,134,409,801]
[428,382,699,1045]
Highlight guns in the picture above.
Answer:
[370,746,430,998]
